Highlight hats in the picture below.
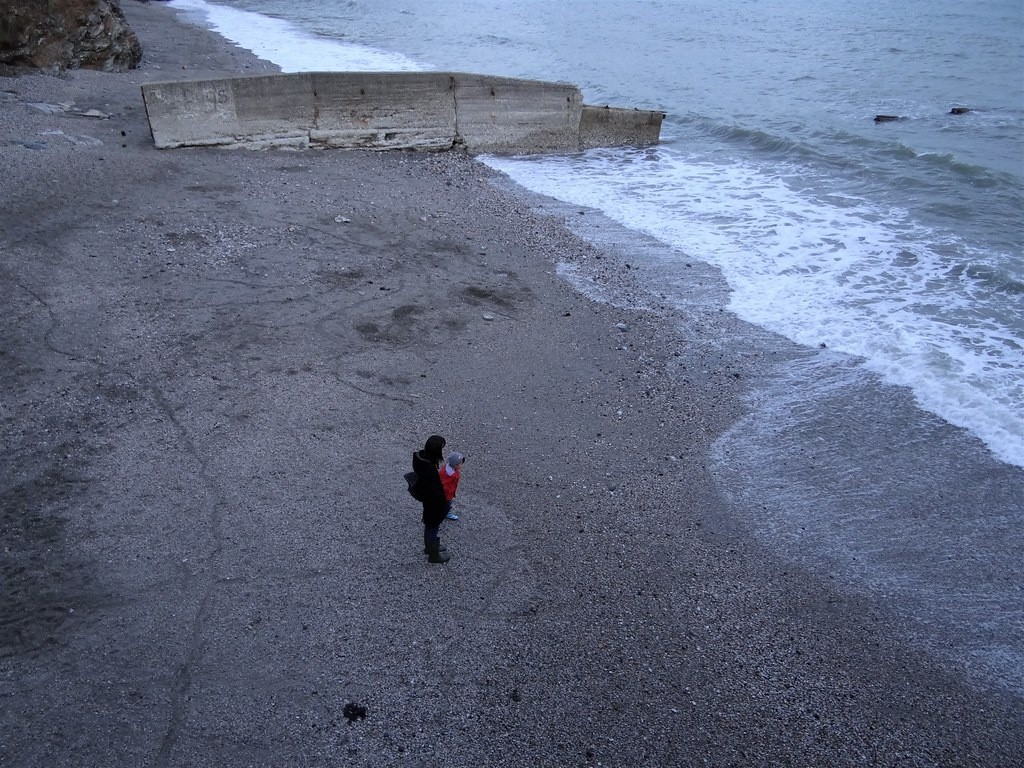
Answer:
[448,452,464,467]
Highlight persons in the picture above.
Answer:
[439,452,465,520]
[412,435,450,562]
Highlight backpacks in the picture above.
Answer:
[404,472,425,503]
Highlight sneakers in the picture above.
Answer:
[445,514,459,521]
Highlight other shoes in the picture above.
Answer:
[423,544,447,554]
[428,553,451,563]
